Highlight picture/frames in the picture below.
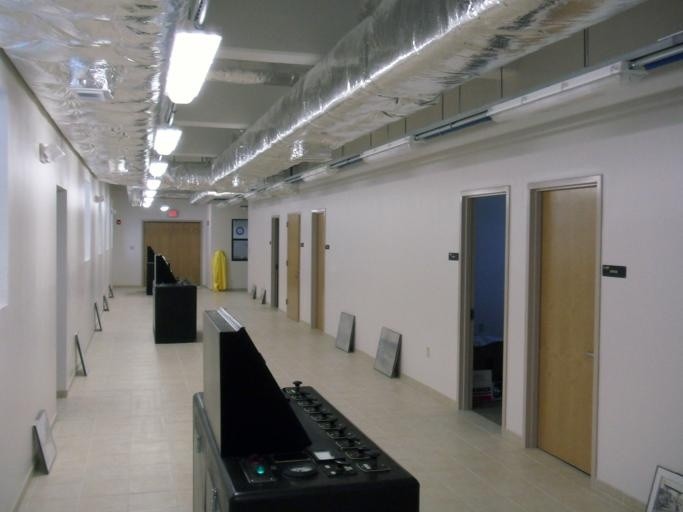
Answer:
[646,465,683,512]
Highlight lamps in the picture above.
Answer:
[164,22,223,105]
[40,142,66,163]
